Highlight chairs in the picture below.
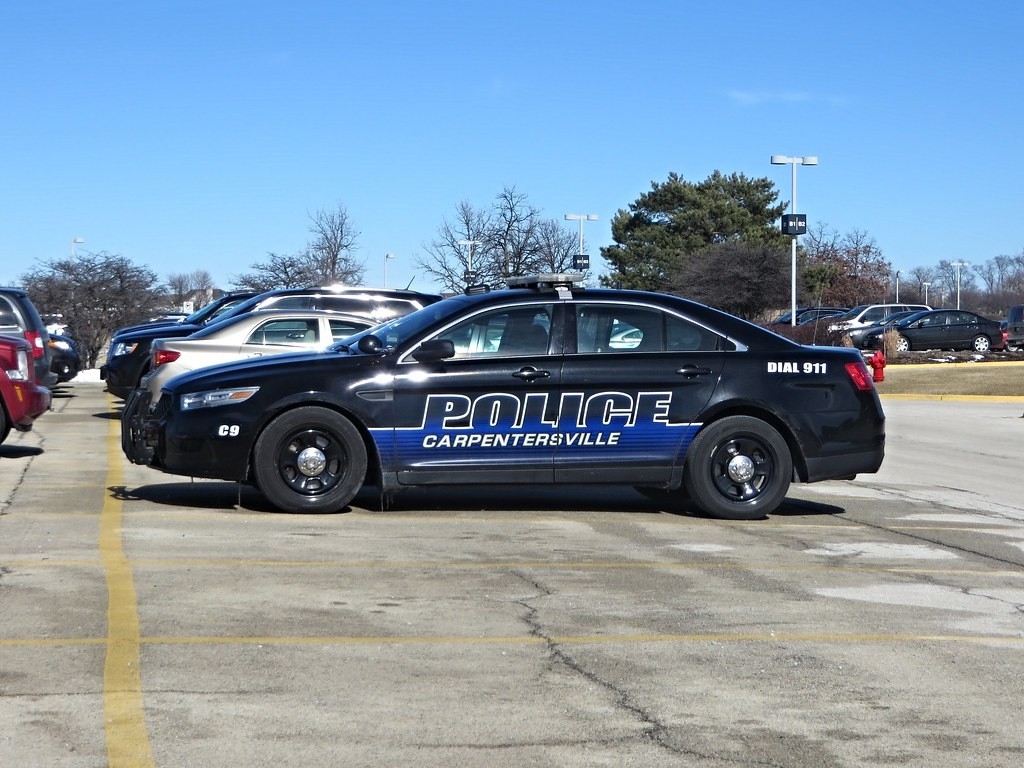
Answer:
[519,323,547,354]
[303,329,316,343]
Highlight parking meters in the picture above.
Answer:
[71,238,85,263]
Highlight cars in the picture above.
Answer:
[843,311,1009,352]
[141,307,456,404]
[119,273,886,521]
[48,333,80,383]
[446,317,643,349]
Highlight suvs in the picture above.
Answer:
[827,303,934,337]
[100,286,445,399]
[0,335,52,445]
[767,307,849,326]
[0,287,58,389]
[1007,304,1024,350]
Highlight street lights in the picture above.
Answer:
[949,262,969,310]
[384,252,395,288]
[923,283,931,305]
[771,155,818,326]
[565,214,598,272]
[460,240,482,273]
